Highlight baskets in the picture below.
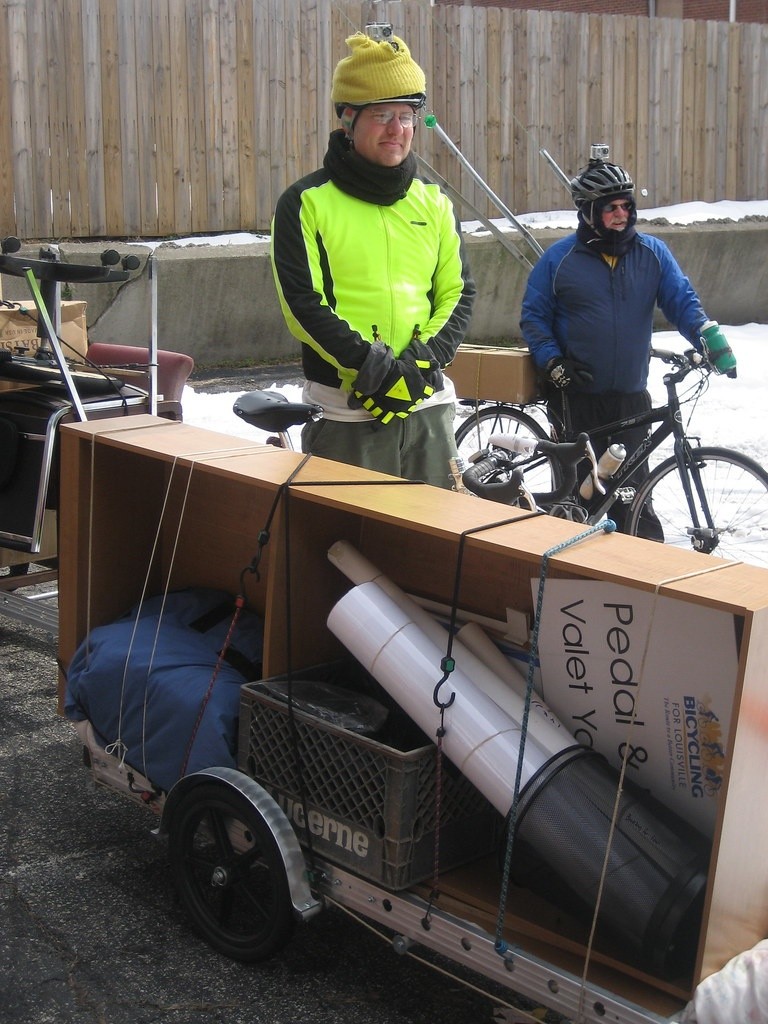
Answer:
[237,662,505,892]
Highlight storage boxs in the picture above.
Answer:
[442,343,540,405]
[0,300,88,393]
[236,657,526,892]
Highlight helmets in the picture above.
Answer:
[571,162,634,212]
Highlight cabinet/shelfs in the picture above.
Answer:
[56,414,767,1024]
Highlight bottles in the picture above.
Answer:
[699,318,738,374]
[580,444,626,499]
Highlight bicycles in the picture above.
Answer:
[232,384,536,509]
[449,347,768,570]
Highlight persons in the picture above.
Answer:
[266,25,476,488]
[519,157,739,543]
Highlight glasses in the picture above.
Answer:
[364,106,421,128]
[604,201,632,212]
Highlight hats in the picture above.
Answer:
[330,32,426,108]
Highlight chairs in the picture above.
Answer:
[0,236,194,554]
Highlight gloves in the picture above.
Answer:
[348,340,445,426]
[545,356,589,395]
